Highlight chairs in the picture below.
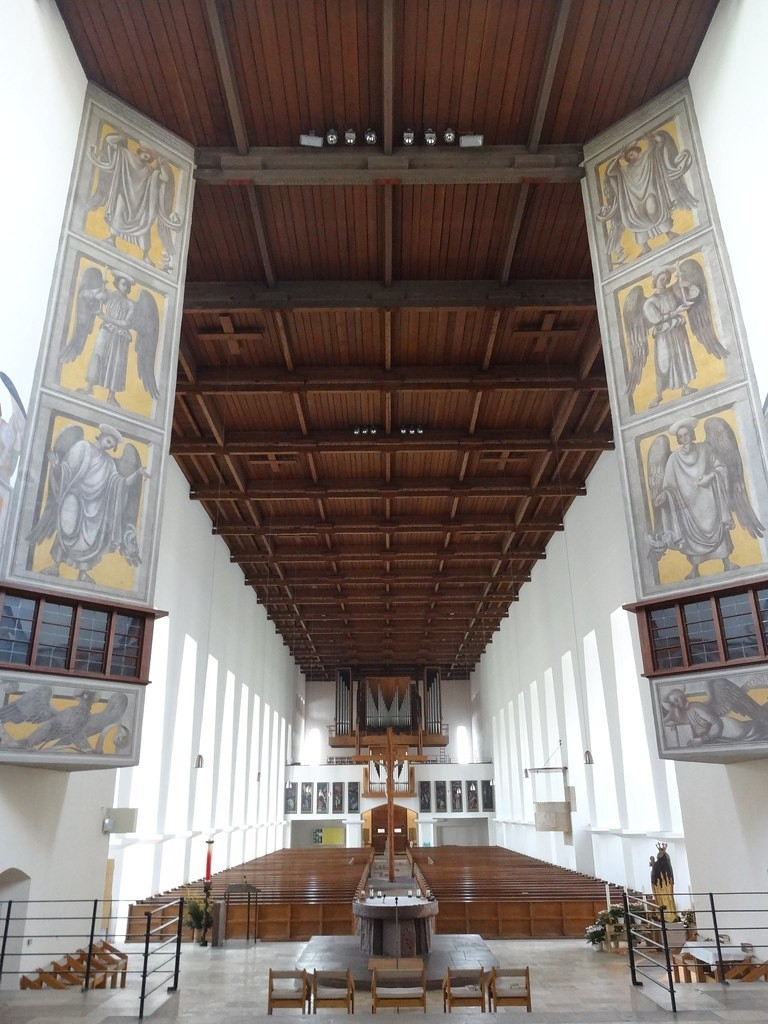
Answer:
[367,958,427,1014]
[20,939,128,990]
[268,968,307,1016]
[486,966,531,1013]
[442,966,486,1013]
[671,935,768,983]
[311,968,355,1015]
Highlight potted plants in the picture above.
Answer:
[184,883,212,948]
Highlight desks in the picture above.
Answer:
[679,940,754,984]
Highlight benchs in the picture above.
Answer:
[125,845,658,945]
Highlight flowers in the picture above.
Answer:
[681,909,697,925]
[628,900,645,917]
[609,932,619,942]
[609,903,626,919]
[584,925,606,946]
[596,909,623,935]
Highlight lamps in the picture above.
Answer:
[545,350,595,767]
[444,128,455,145]
[403,128,414,146]
[365,127,377,144]
[326,129,339,145]
[191,336,239,767]
[424,129,438,146]
[344,129,357,145]
[459,132,484,147]
[299,130,324,147]
[351,424,424,438]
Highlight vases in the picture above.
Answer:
[618,917,625,924]
[606,925,615,941]
[683,923,699,941]
[633,917,642,925]
[592,942,604,952]
[610,939,619,948]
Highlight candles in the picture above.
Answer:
[408,890,412,895]
[417,889,420,896]
[426,889,430,897]
[361,890,365,898]
[378,891,381,896]
[369,889,374,896]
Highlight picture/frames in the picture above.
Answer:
[347,782,360,813]
[0,669,146,771]
[0,80,198,609]
[332,782,345,815]
[282,782,297,815]
[316,782,330,814]
[465,780,480,812]
[449,780,463,812]
[649,663,768,754]
[301,782,314,814]
[417,781,432,813]
[481,779,496,813]
[433,781,448,813]
[580,78,768,601]
